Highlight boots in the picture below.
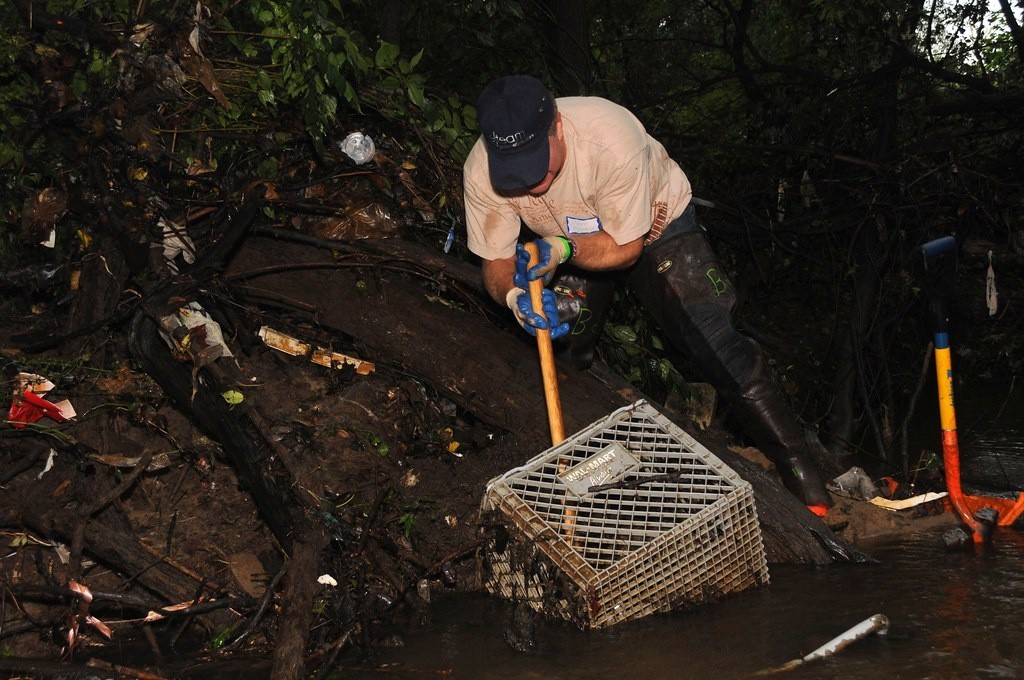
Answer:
[741,379,832,517]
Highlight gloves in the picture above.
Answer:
[507,286,570,338]
[515,236,575,291]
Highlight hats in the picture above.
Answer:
[477,75,555,191]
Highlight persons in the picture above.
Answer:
[464,73,831,519]
